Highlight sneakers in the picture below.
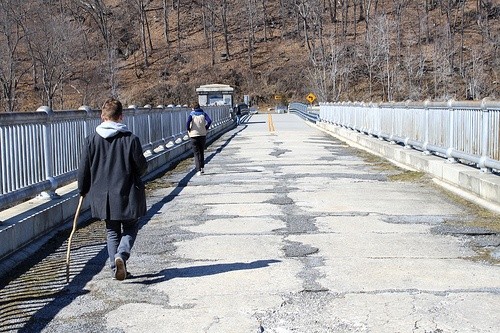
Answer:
[112,257,132,280]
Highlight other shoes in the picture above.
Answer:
[197,168,204,176]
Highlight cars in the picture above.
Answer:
[235,102,248,115]
[277,105,288,112]
[249,107,259,114]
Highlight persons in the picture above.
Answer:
[76,95,148,281]
[236,104,241,126]
[186,101,212,175]
